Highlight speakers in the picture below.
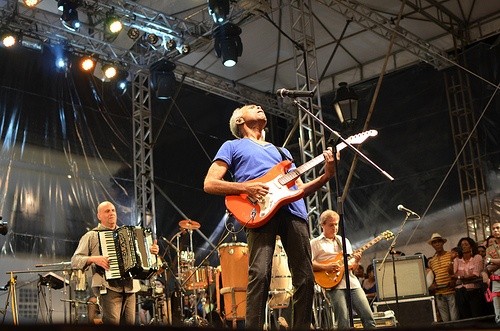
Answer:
[372,253,429,302]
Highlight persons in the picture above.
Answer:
[352,219,500,328]
[70,200,159,331]
[86,278,213,331]
[203,105,340,331]
[309,209,378,331]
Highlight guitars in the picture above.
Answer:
[224,129,378,229]
[313,230,395,289]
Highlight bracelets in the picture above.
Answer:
[487,257,490,264]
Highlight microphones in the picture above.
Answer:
[276,87,314,98]
[230,223,237,244]
[397,204,418,217]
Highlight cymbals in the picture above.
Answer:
[178,219,201,229]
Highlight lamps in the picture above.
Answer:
[100,63,128,93]
[145,33,158,44]
[56,0,81,32]
[127,28,140,39]
[208,0,233,24]
[332,81,359,124]
[213,22,243,67]
[3,33,16,48]
[177,40,191,55]
[149,59,178,101]
[106,12,123,34]
[162,36,176,51]
[80,56,95,71]
[41,44,71,73]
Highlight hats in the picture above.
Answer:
[428,233,447,244]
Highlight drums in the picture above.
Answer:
[268,235,293,309]
[201,266,214,286]
[217,242,248,321]
[184,266,207,291]
